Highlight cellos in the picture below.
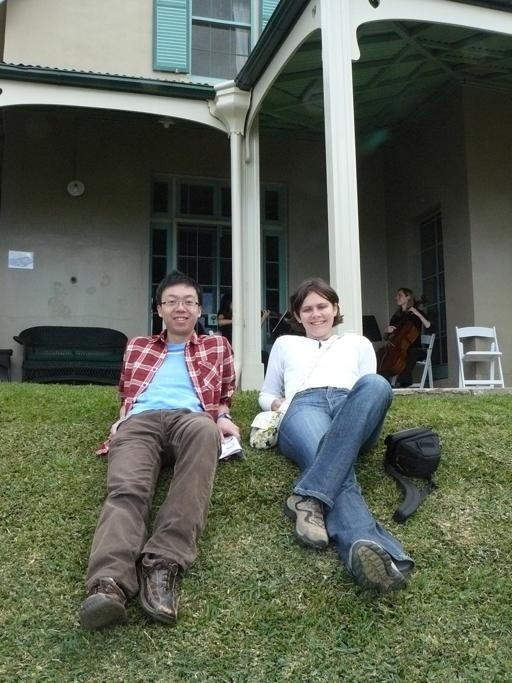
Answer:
[376,295,427,377]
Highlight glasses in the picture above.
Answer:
[159,297,201,307]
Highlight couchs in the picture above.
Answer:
[13,326,128,385]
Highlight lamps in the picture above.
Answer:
[158,116,177,130]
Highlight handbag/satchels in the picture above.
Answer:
[244,408,289,452]
[379,417,449,527]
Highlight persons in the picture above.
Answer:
[256,277,416,597]
[77,269,241,636]
[217,291,272,378]
[383,286,434,387]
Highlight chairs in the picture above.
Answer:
[407,333,436,387]
[455,326,506,390]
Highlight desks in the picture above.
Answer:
[0,349,13,383]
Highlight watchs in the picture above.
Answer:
[216,413,233,422]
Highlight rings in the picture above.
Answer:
[392,328,393,331]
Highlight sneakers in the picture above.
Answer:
[136,550,190,628]
[74,572,134,635]
[281,486,335,552]
[343,536,413,597]
